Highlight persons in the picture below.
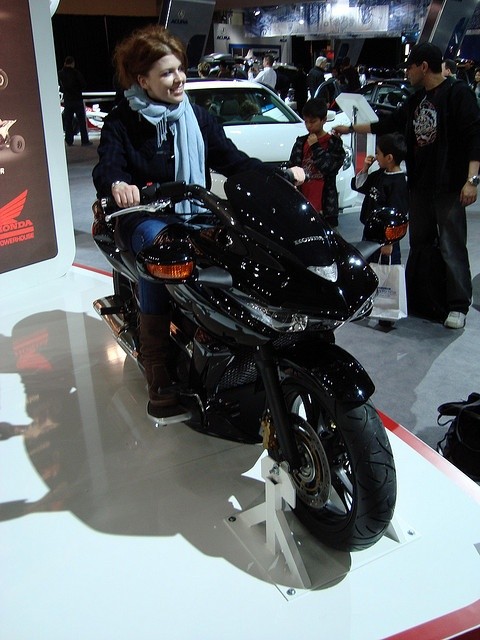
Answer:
[288,99,346,235]
[248,55,277,89]
[59,56,91,145]
[351,132,414,329]
[356,63,367,90]
[339,57,361,92]
[228,101,259,123]
[198,62,211,79]
[193,93,214,111]
[441,58,460,78]
[333,44,480,329]
[93,29,305,410]
[470,70,480,107]
[218,62,230,78]
[307,56,328,98]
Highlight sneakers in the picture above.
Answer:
[444,310,466,329]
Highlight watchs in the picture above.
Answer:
[468,174,480,188]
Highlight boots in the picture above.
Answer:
[138,314,181,408]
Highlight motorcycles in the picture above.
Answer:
[93,164,411,551]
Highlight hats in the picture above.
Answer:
[398,43,441,68]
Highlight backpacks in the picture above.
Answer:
[436,392,480,481]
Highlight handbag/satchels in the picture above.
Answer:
[369,253,408,321]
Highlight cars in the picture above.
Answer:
[182,76,359,210]
[356,80,412,118]
